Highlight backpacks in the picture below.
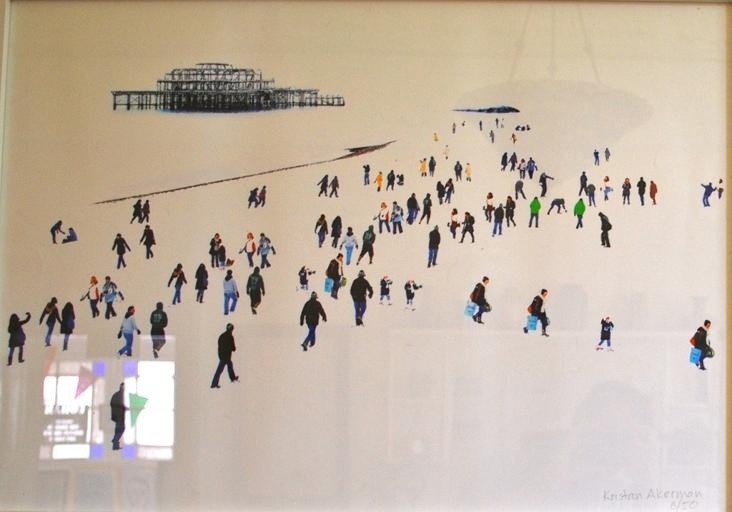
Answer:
[248,275,260,292]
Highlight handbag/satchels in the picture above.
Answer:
[113,289,125,305]
[602,219,611,231]
[322,260,348,294]
[526,296,548,333]
[464,285,492,320]
[688,327,714,367]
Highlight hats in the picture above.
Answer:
[602,314,611,321]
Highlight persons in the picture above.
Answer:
[694,320,711,370]
[111,383,130,450]
[701,182,716,207]
[718,179,723,198]
[211,324,238,388]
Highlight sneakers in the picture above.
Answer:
[302,342,315,351]
